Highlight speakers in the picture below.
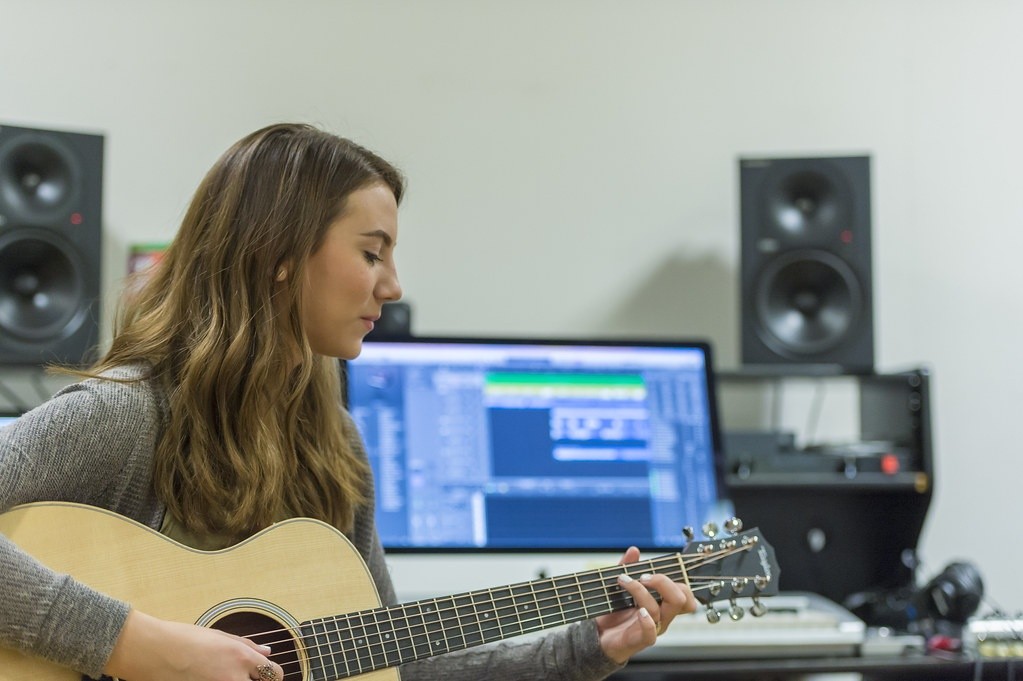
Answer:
[0,125,105,367]
[737,156,874,376]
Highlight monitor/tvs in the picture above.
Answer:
[343,335,726,607]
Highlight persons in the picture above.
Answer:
[1,122,697,681]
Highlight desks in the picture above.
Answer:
[376,551,1023,681]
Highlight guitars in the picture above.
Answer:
[1,497,784,680]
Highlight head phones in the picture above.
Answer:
[873,562,985,632]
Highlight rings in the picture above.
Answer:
[255,661,281,681]
